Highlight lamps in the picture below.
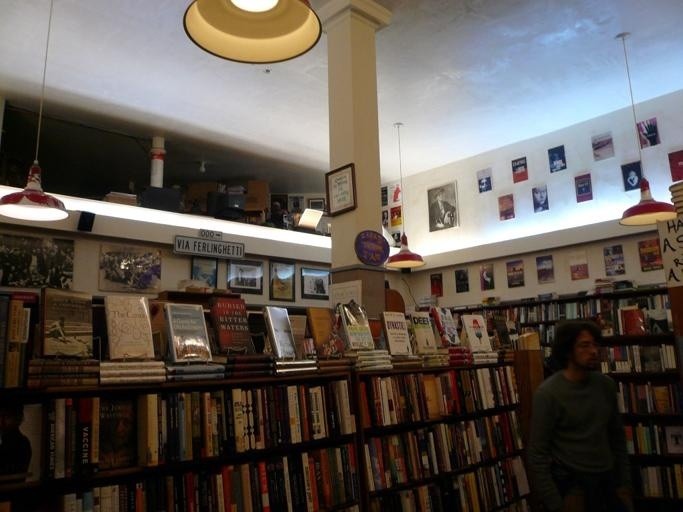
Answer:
[385,122,425,270]
[182,1,323,64]
[0,0,70,222]
[612,30,678,227]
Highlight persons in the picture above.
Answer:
[429,187,457,229]
[532,185,549,212]
[479,179,491,191]
[100,249,162,289]
[638,119,659,147]
[1,244,75,290]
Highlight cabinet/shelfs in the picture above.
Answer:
[450,287,683,511]
[0,362,546,511]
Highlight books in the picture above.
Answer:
[1,278,683,511]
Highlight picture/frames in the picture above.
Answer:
[324,162,358,218]
[191,256,330,303]
